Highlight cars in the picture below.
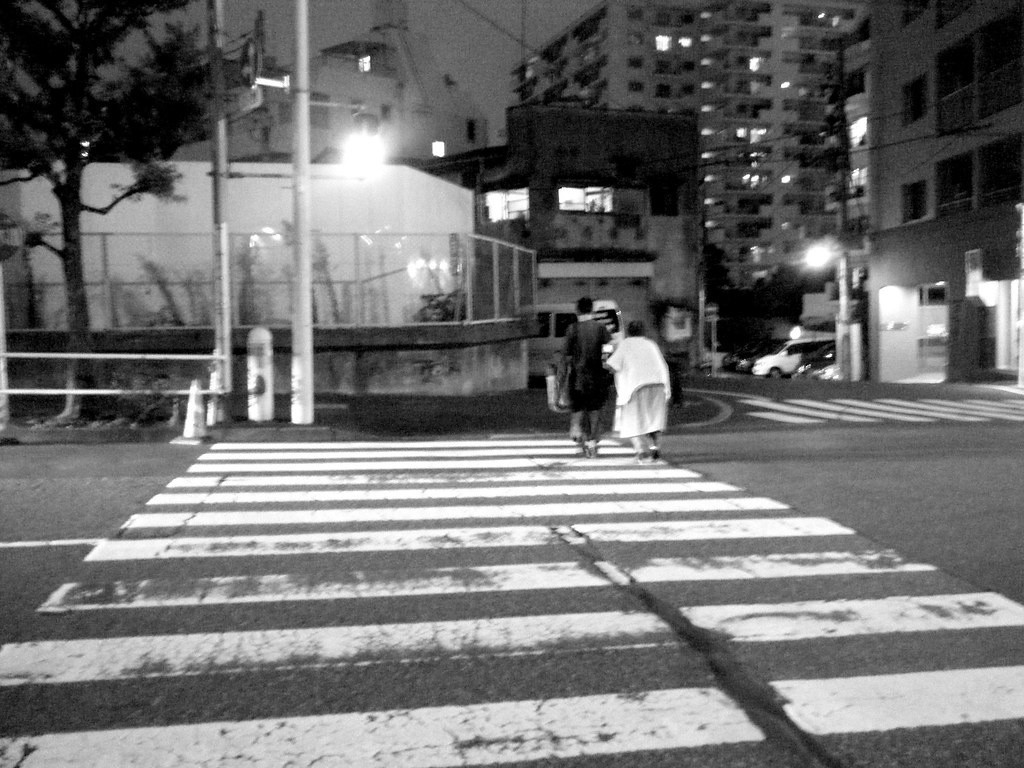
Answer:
[752,339,834,380]
[736,347,772,373]
[791,342,835,380]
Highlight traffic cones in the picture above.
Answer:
[170,380,213,445]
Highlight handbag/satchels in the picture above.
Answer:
[554,358,573,409]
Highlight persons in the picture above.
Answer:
[553,297,613,458]
[606,321,672,462]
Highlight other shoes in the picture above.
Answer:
[649,445,659,461]
[635,450,644,462]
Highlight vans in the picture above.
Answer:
[522,300,626,376]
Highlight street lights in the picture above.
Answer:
[820,49,851,385]
